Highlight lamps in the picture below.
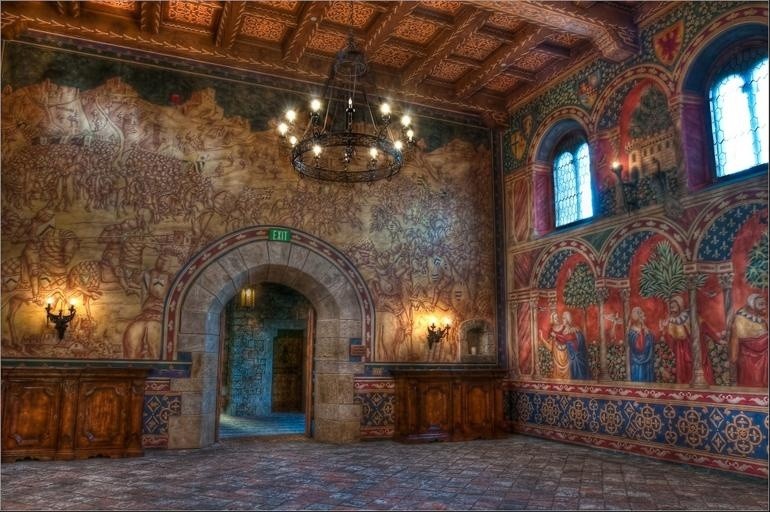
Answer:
[43,291,78,339]
[609,161,634,215]
[238,284,255,313]
[425,314,453,348]
[273,1,419,185]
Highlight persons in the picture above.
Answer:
[658,296,727,386]
[729,293,768,388]
[539,312,570,379]
[551,311,594,379]
[628,307,656,382]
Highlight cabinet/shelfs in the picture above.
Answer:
[0,366,154,463]
[390,364,509,443]
